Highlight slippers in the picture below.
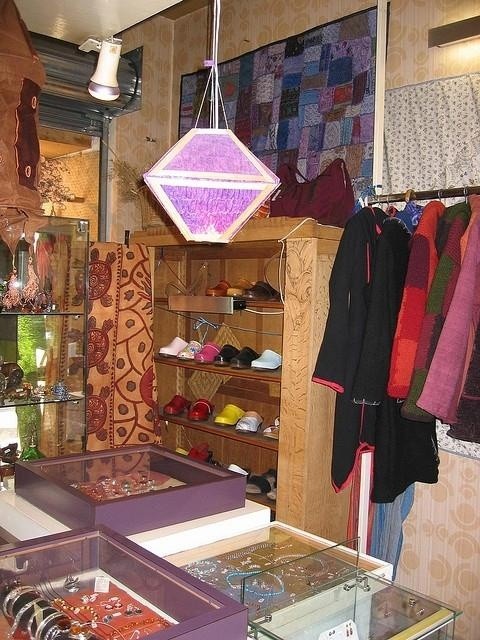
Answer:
[249,348,281,372]
[186,397,218,422]
[196,341,222,365]
[234,411,266,434]
[206,278,232,297]
[262,417,280,439]
[214,404,246,427]
[242,281,281,301]
[213,343,241,368]
[157,336,190,359]
[231,346,262,370]
[175,340,203,362]
[162,394,191,417]
[226,277,255,297]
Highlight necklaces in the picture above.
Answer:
[182,535,330,597]
[34,580,118,640]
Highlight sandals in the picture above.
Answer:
[186,442,213,463]
[245,467,276,494]
[228,463,251,478]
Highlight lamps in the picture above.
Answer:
[142,0,282,245]
[77,35,123,102]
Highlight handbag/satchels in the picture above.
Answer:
[269,156,355,228]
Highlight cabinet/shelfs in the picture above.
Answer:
[128,220,351,549]
[1,212,88,491]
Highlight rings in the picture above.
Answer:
[83,468,155,498]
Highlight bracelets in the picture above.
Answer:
[2,585,94,640]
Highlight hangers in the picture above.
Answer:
[342,185,480,236]
